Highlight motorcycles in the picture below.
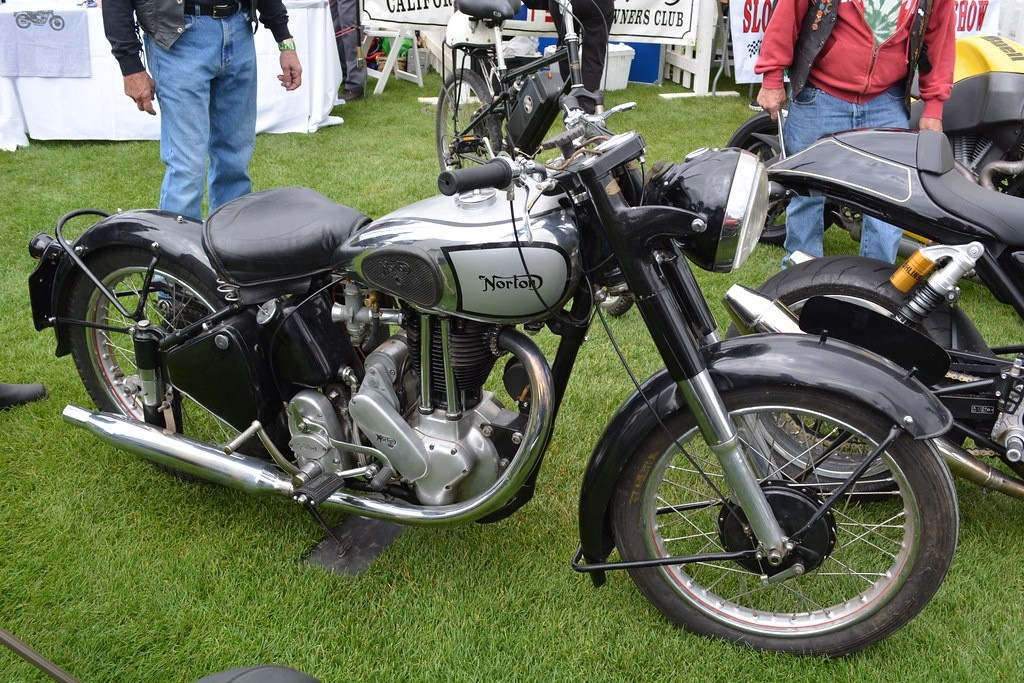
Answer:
[27,80,961,659]
[724,27,1024,312]
[720,70,1024,501]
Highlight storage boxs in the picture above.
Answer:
[407,47,430,76]
[544,43,635,91]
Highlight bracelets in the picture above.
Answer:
[278,38,296,50]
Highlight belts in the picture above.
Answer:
[183,3,242,19]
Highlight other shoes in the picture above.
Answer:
[338,88,362,102]
[0,380,47,407]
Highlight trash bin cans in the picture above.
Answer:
[492,55,543,86]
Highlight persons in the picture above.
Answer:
[754,0,956,267]
[328,0,365,102]
[101,1,302,309]
[548,0,614,114]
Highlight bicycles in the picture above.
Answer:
[436,0,603,170]
